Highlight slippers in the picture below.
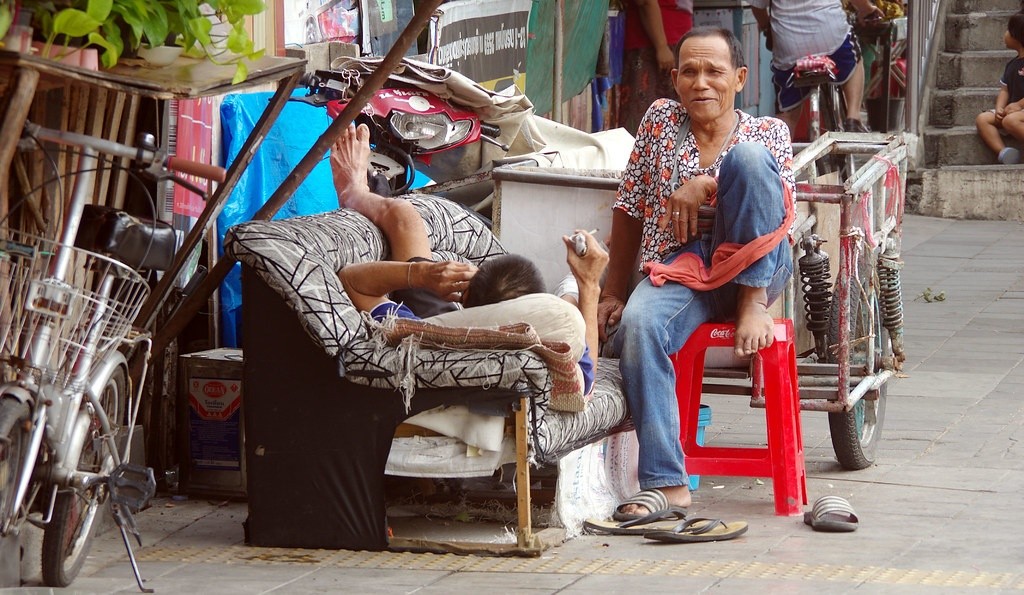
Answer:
[612,486,689,523]
[804,494,860,533]
[584,509,750,543]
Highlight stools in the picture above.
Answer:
[669,317,810,516]
[178,348,246,497]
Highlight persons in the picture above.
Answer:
[625,0,694,138]
[977,10,1024,164]
[597,26,797,520]
[330,122,611,394]
[747,0,885,143]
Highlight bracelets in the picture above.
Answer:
[408,261,415,287]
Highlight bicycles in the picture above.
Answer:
[1,118,228,593]
[763,9,880,184]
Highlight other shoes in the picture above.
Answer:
[997,147,1024,165]
[845,118,870,133]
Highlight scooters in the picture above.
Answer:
[269,68,509,195]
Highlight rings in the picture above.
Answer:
[672,211,680,215]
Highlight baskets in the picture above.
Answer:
[0,224,152,394]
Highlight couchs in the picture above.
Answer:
[222,192,639,559]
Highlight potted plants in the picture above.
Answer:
[49,0,269,85]
